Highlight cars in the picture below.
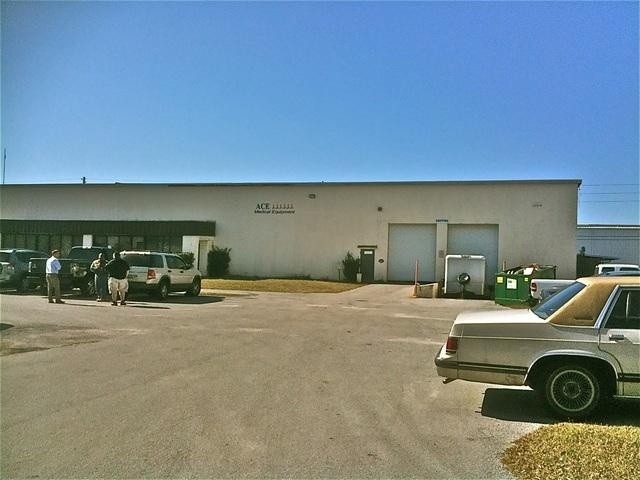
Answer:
[433,273,640,418]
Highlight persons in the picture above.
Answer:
[90,253,109,302]
[45,250,65,303]
[105,253,130,306]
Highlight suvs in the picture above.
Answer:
[113,249,202,301]
[0,249,52,291]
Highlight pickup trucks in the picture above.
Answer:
[530,262,640,303]
[28,245,119,297]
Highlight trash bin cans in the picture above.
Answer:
[494,264,557,304]
